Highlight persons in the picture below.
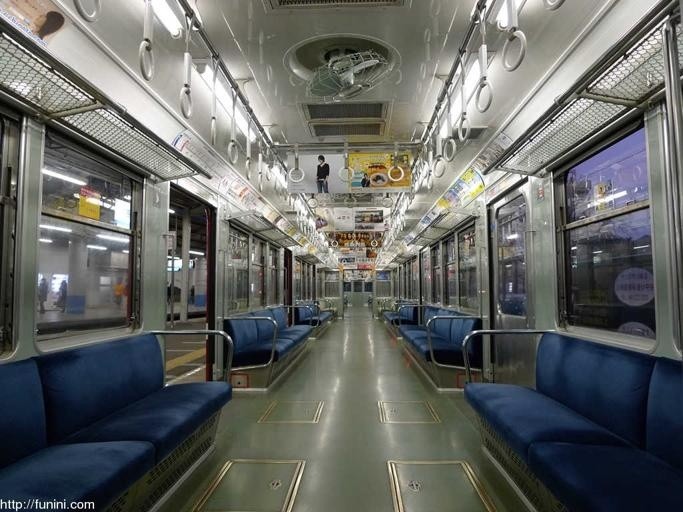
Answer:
[114,277,125,304]
[191,285,194,303]
[361,173,370,187]
[32,11,64,40]
[39,278,48,314]
[57,280,67,313]
[316,155,329,193]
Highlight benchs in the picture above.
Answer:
[462,330,681,511]
[0,334,232,511]
[222,301,339,393]
[378,298,484,392]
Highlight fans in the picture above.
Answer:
[302,44,397,108]
